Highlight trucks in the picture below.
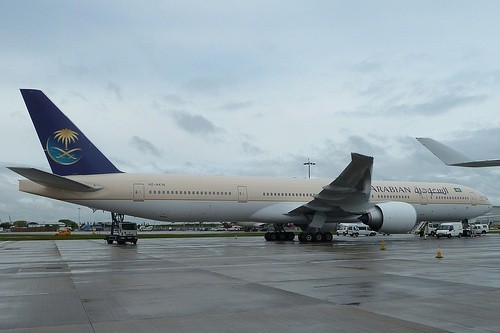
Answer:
[105,222,141,246]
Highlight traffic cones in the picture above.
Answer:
[435,239,443,258]
[380,238,386,250]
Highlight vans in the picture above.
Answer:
[435,222,463,238]
[471,223,490,234]
[347,224,377,237]
[336,223,349,236]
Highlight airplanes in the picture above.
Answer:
[5,87,495,246]
